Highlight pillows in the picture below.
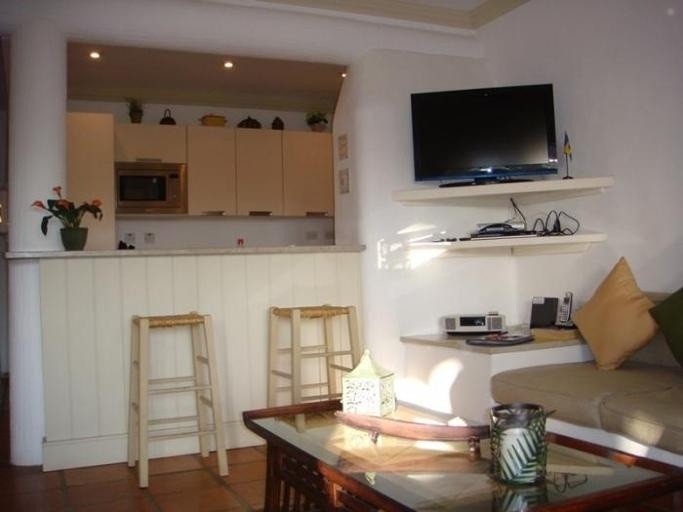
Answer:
[649,286,683,367]
[570,258,658,370]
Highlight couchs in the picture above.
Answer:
[489,291,682,476]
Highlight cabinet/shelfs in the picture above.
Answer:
[67,113,115,251]
[116,122,186,165]
[188,127,284,221]
[400,324,593,421]
[284,130,334,218]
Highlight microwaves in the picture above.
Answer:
[117,168,180,211]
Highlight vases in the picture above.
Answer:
[60,227,87,249]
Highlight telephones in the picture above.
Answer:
[555,290,579,327]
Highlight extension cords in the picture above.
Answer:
[537,229,562,235]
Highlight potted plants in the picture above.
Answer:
[127,96,143,123]
[306,111,328,131]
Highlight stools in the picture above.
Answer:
[268,304,361,405]
[127,312,229,489]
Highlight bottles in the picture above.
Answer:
[270,116,283,130]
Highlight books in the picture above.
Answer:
[467,331,536,347]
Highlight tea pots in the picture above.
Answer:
[159,107,175,125]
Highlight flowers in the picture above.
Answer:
[31,186,103,236]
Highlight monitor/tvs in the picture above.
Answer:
[410,84,558,187]
